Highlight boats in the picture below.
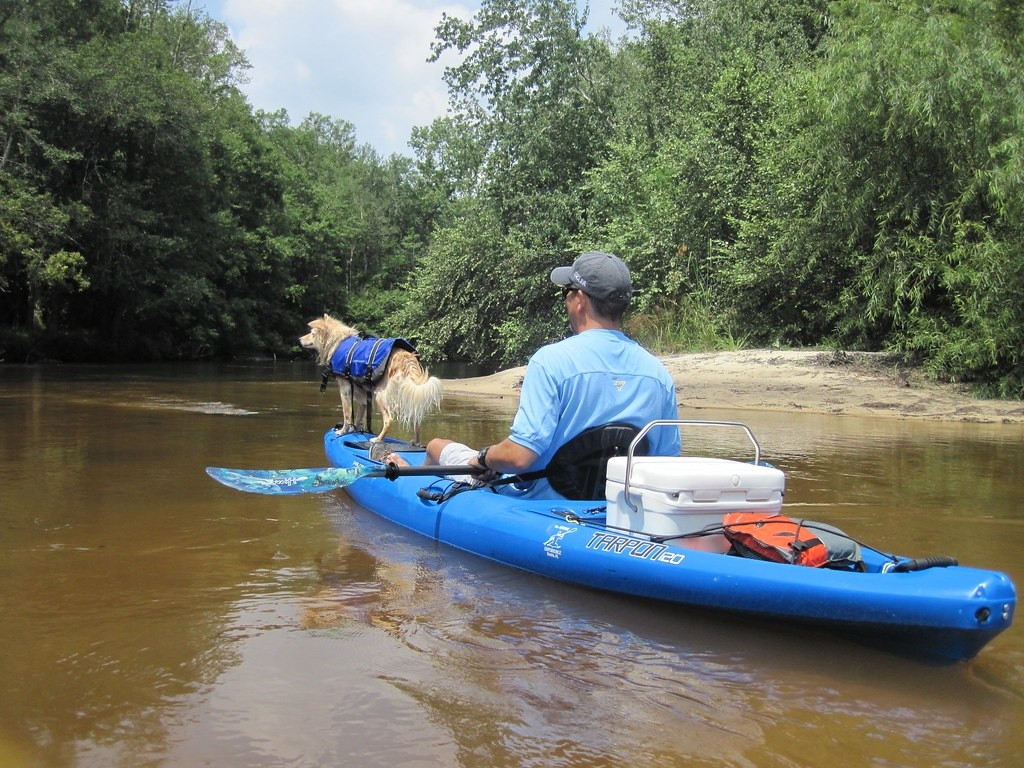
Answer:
[323,419,1016,654]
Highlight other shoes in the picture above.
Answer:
[370,442,393,464]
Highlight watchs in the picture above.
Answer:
[476,446,491,471]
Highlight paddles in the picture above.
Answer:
[206,464,484,497]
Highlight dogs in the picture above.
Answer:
[298,313,443,446]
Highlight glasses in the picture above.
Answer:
[562,287,590,299]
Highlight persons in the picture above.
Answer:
[385,251,681,501]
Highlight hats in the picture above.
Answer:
[550,251,632,303]
